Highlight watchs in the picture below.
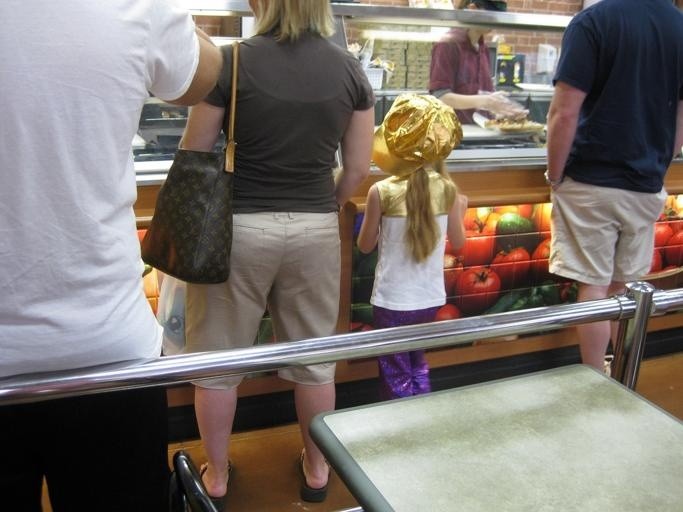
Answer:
[542,169,564,188]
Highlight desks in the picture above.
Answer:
[309,363,683,509]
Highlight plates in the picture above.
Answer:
[515,84,553,93]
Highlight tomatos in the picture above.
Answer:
[352,193,683,350]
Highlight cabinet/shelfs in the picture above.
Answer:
[331,1,591,170]
[122,3,268,182]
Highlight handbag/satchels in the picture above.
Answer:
[142,149,234,284]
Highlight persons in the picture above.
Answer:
[543,0,682,377]
[0,0,226,511]
[355,89,470,401]
[179,1,376,512]
[427,0,532,127]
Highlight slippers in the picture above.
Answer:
[299,448,329,502]
[201,458,231,505]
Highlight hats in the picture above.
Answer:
[373,95,462,177]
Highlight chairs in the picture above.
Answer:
[167,453,222,512]
